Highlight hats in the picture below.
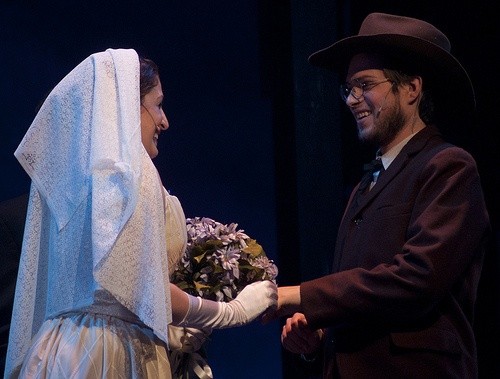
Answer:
[307,13,476,111]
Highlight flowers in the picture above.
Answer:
[169,217,279,379]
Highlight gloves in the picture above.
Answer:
[167,324,211,355]
[178,278,279,330]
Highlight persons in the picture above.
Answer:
[278,13,500,379]
[3,48,278,379]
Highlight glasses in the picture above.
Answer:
[340,79,388,101]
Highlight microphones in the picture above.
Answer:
[377,82,396,113]
[141,102,161,133]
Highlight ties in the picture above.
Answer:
[349,158,385,208]
